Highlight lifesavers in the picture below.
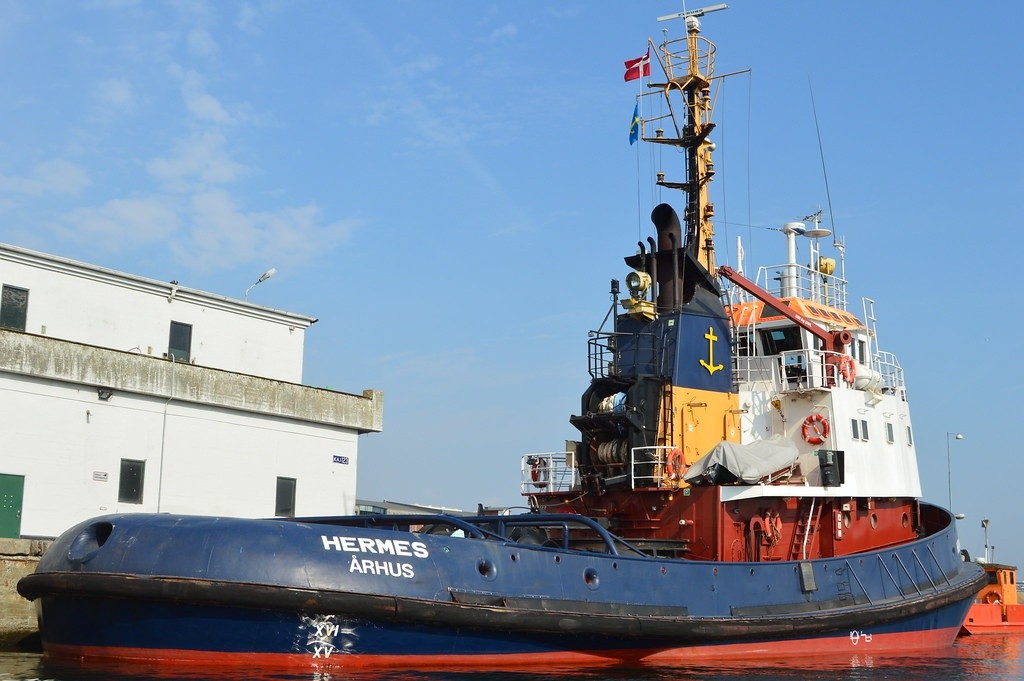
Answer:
[764,508,781,541]
[667,448,686,479]
[803,413,830,445]
[840,354,857,383]
[532,457,551,489]
[986,591,1003,605]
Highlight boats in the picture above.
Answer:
[18,0,988,669]
[946,431,1024,635]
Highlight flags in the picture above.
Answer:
[623,52,651,82]
[629,100,639,145]
